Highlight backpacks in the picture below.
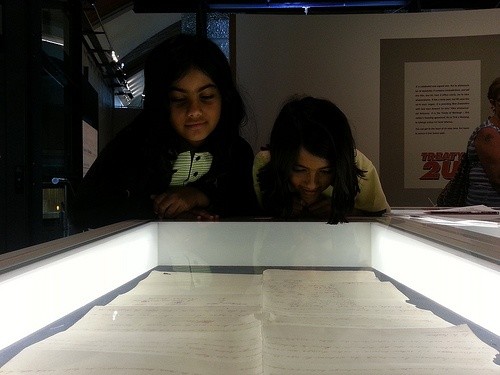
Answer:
[437,152,469,207]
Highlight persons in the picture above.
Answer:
[459,77,500,208]
[68,32,255,231]
[250,95,392,225]
